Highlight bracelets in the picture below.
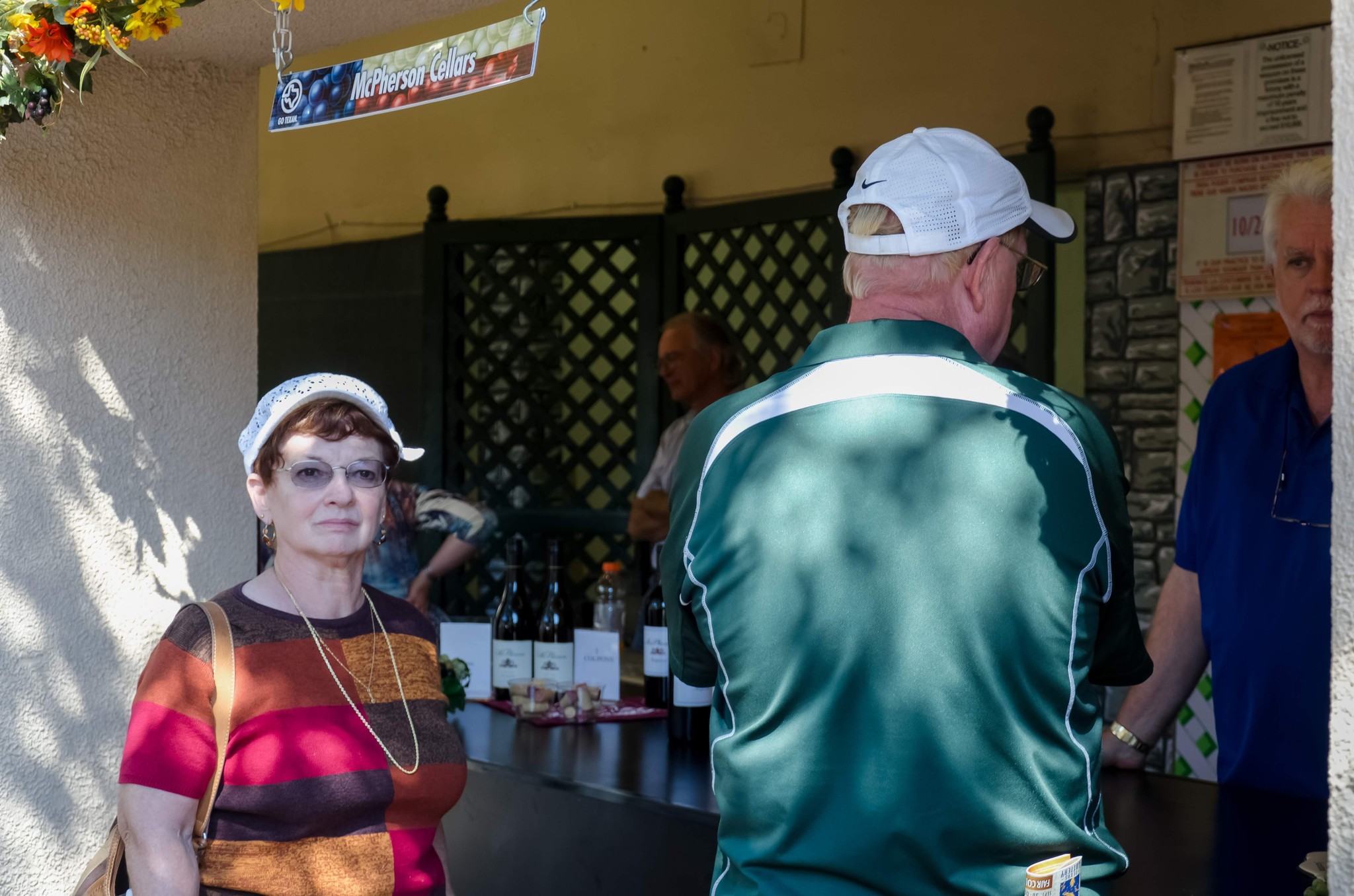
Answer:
[425,567,439,584]
[1110,720,1152,757]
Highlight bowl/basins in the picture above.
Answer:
[507,679,557,716]
[555,682,605,716]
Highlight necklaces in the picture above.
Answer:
[275,571,420,773]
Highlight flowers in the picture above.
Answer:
[0,1,205,141]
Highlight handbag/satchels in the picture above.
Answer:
[69,814,124,896]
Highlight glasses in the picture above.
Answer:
[968,238,1048,290]
[269,459,397,488]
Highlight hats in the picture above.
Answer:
[238,372,425,476]
[837,128,1077,256]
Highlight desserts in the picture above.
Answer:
[509,679,601,722]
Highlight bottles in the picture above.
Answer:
[534,541,578,683]
[594,564,626,663]
[491,538,533,701]
[642,570,673,709]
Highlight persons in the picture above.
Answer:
[361,478,500,636]
[628,314,739,579]
[657,128,1153,896]
[116,373,467,896]
[1103,156,1335,794]
